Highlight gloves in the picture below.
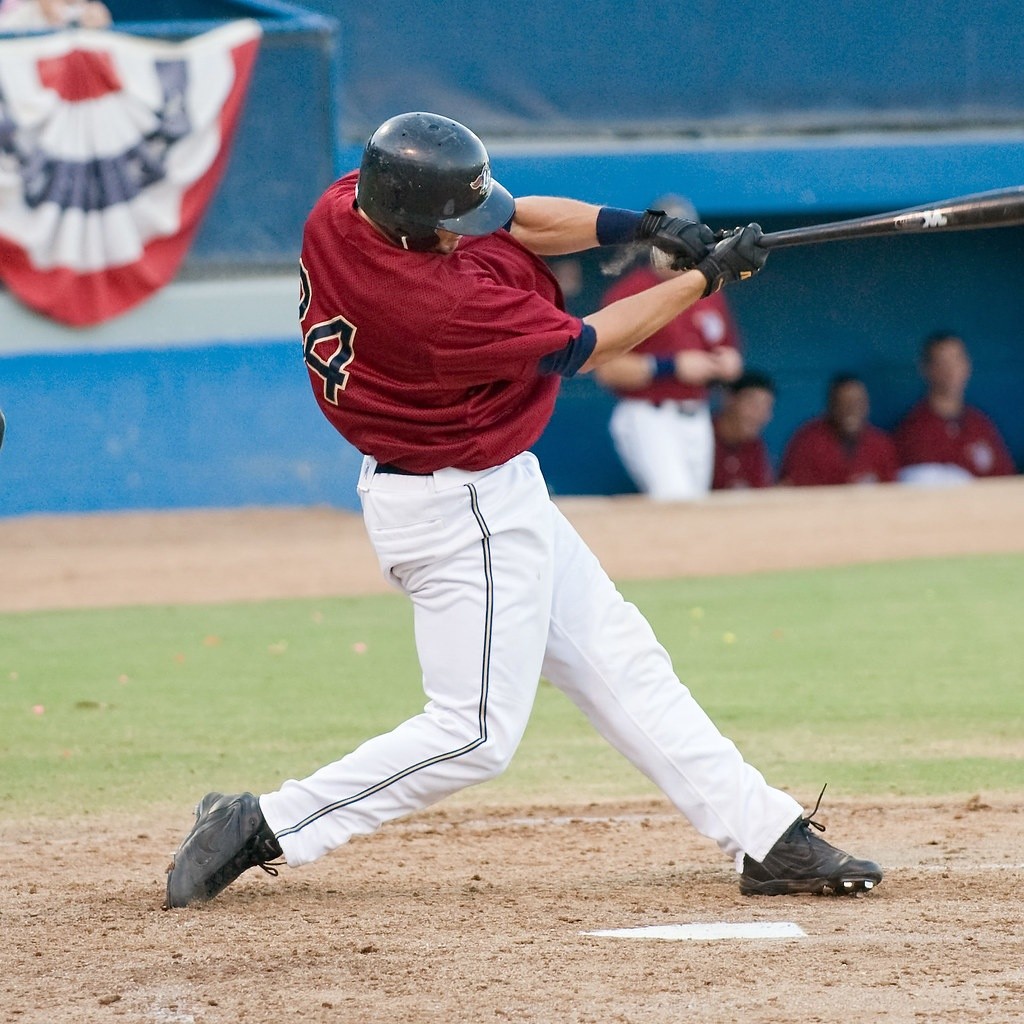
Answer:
[637,209,718,271]
[693,222,770,300]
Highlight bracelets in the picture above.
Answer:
[596,207,647,246]
[650,351,675,379]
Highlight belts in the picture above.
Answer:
[364,462,434,476]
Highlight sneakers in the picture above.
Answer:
[165,791,288,910]
[739,783,883,897]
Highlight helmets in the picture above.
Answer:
[355,111,516,251]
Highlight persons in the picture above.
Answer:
[166,113,882,908]
[602,192,1011,498]
[0,0,111,31]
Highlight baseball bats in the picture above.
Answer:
[651,187,1024,272]
